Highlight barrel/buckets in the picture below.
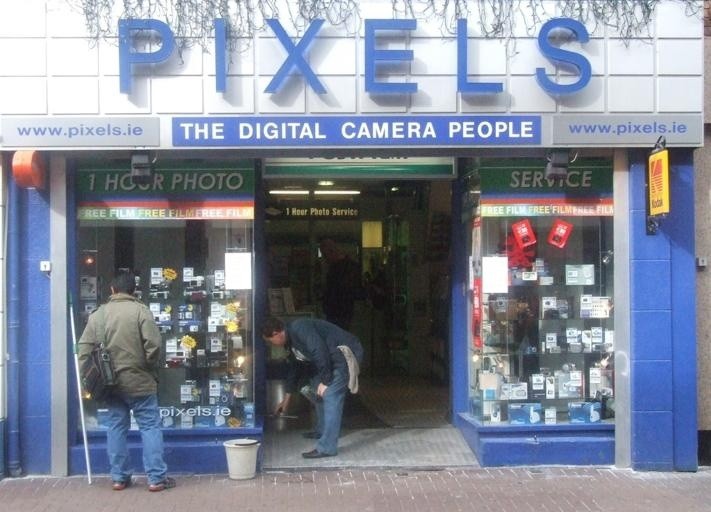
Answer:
[223,438,261,479]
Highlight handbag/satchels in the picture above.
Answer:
[81,340,120,401]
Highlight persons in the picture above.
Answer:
[77,270,176,493]
[257,316,367,458]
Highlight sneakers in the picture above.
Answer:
[148,476,175,490]
[112,480,130,489]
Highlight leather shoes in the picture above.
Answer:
[304,432,322,439]
[303,450,338,458]
